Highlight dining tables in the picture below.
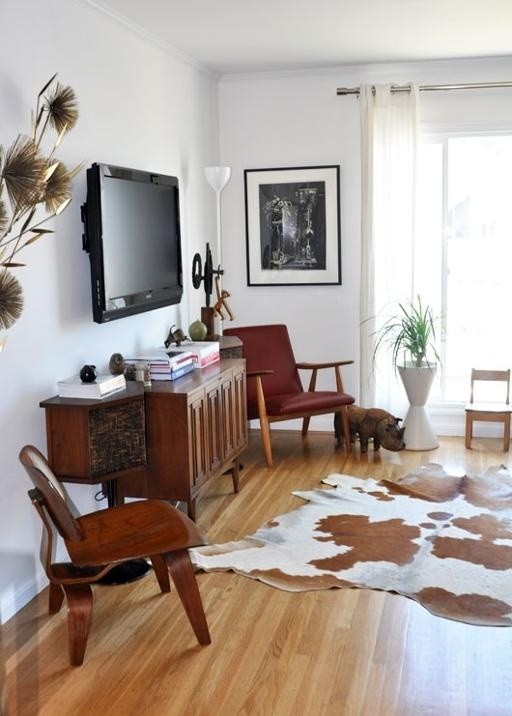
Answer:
[358,296,446,452]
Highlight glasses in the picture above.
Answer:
[204,166,233,335]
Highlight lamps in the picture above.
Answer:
[223,324,355,469]
[19,446,212,666]
[464,368,512,451]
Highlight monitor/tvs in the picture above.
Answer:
[124,349,194,380]
[55,372,126,400]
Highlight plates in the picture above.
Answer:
[38,358,249,522]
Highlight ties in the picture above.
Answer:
[86,162,183,323]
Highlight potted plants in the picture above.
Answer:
[244,164,342,286]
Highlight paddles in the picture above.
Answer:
[144,462,511,627]
